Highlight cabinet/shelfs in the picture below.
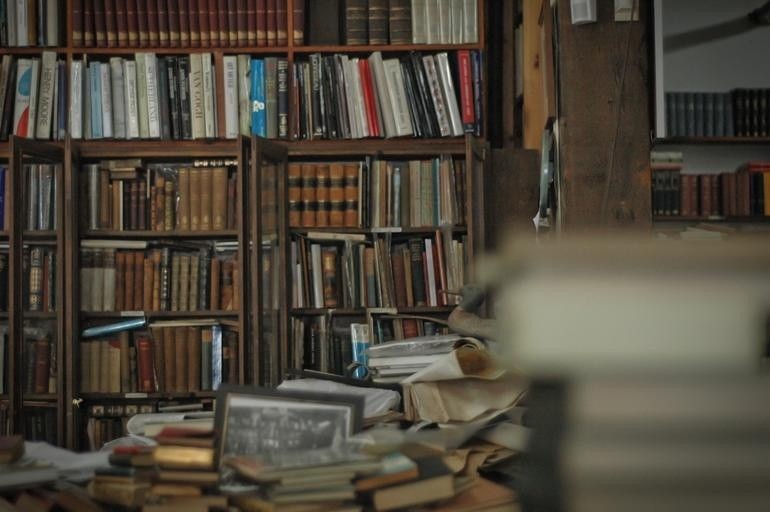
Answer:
[244,139,491,511]
[0,137,247,511]
[486,0,770,512]
[0,0,485,138]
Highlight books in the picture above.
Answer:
[1,1,530,512]
[470,219,770,512]
[665,88,769,137]
[650,151,769,217]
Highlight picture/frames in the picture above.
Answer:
[210,383,365,473]
